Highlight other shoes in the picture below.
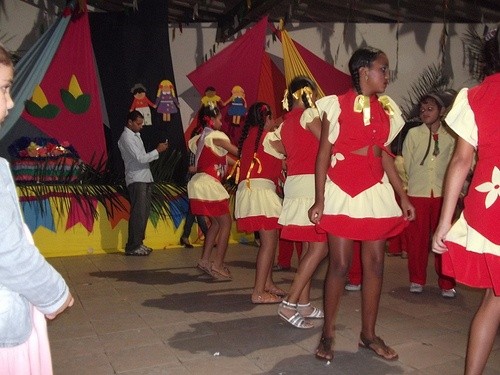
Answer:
[443,288,457,296]
[197,259,232,282]
[410,283,423,293]
[273,265,282,271]
[251,292,282,303]
[267,284,287,296]
[345,284,360,291]
[180,237,193,248]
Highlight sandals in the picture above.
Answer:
[278,299,313,329]
[359,333,398,362]
[315,335,335,361]
[297,303,324,322]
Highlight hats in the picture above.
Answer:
[426,88,457,114]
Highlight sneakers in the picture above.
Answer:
[126,245,153,256]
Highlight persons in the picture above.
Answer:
[118,110,169,257]
[263,74,324,328]
[402,93,476,297]
[235,102,288,304]
[0,43,74,375]
[308,45,416,362]
[432,25,500,375]
[187,101,242,280]
[180,116,470,291]
